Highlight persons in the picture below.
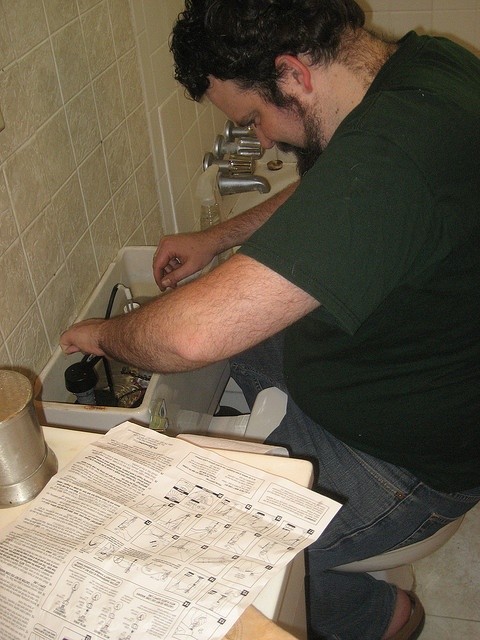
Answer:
[59,2,480,640]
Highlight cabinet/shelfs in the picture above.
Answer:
[1,425,315,640]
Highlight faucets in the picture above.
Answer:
[203,153,270,196]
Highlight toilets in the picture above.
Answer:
[31,245,467,592]
